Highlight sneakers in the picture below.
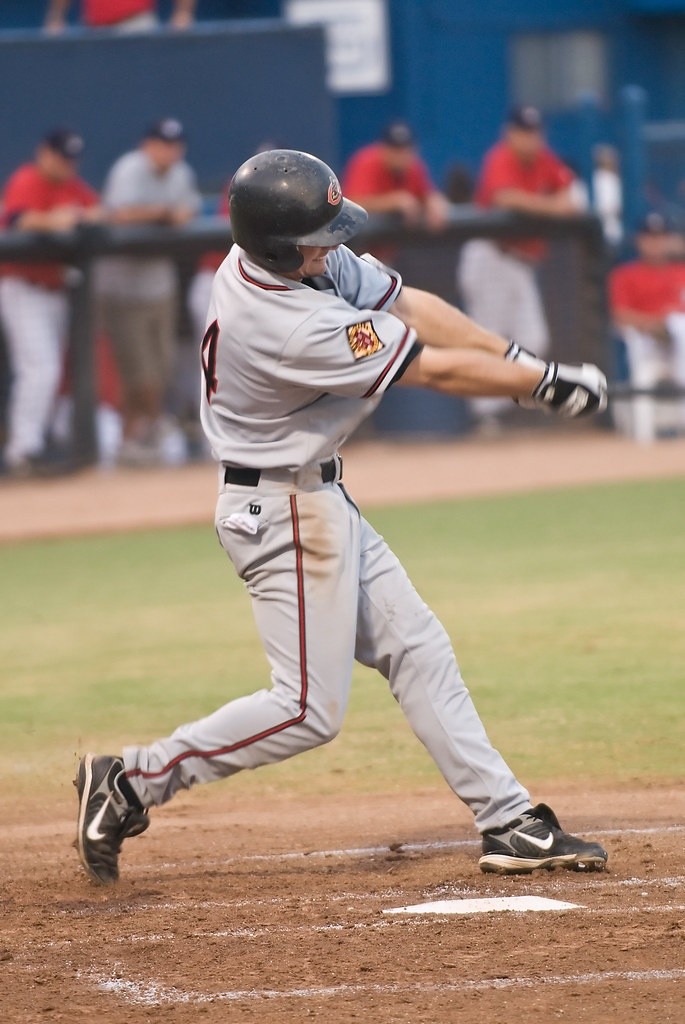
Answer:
[73,753,150,887]
[479,803,608,875]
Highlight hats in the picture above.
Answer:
[634,208,675,238]
[44,126,87,160]
[379,121,412,149]
[142,114,186,143]
[509,106,542,132]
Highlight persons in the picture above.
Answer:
[0,0,685,479]
[71,149,609,883]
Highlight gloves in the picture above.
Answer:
[531,360,608,420]
[503,339,550,409]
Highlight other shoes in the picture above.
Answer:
[117,444,151,466]
[160,416,188,467]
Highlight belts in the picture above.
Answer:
[224,456,343,488]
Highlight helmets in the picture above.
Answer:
[228,149,369,273]
